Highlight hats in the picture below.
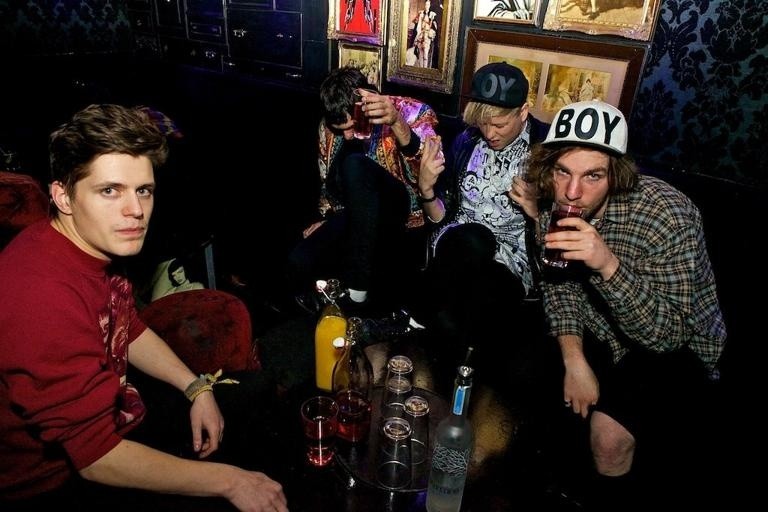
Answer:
[462,62,529,109]
[540,98,630,154]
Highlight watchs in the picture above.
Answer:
[415,192,436,203]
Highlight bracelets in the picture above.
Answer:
[183,366,212,403]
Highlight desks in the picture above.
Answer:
[199,281,583,512]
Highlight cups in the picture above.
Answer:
[540,201,585,270]
[377,415,413,493]
[301,395,337,464]
[349,89,379,140]
[403,395,428,465]
[381,357,414,419]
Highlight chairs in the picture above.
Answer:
[0,174,255,381]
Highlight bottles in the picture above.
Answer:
[312,277,350,386]
[426,365,477,511]
[330,316,375,443]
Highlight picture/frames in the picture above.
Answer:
[543,0,662,45]
[468,0,546,29]
[324,0,389,46]
[458,27,650,127]
[385,0,463,95]
[336,41,383,92]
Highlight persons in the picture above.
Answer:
[300,65,443,319]
[463,97,730,512]
[0,103,347,512]
[411,1,438,67]
[417,60,551,388]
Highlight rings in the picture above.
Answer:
[562,399,571,406]
